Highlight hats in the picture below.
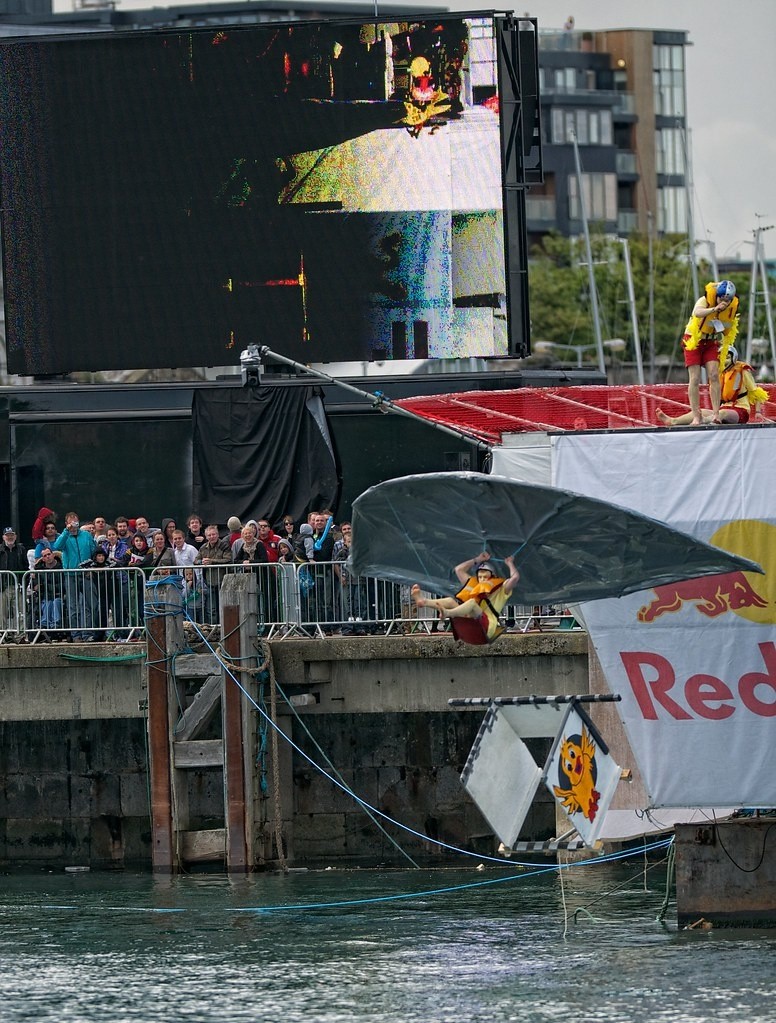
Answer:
[3,527,16,535]
[300,524,313,536]
[96,535,106,543]
[227,516,241,530]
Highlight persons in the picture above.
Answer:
[681,281,740,425]
[0,507,451,644]
[410,552,520,645]
[656,344,769,427]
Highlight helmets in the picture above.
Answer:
[716,280,735,302]
[718,345,738,365]
[477,562,497,578]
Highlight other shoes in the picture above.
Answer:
[19,637,31,644]
[299,624,420,635]
[73,635,95,643]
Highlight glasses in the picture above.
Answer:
[45,527,55,530]
[44,553,50,557]
[99,540,107,544]
[284,523,294,525]
[260,525,269,527]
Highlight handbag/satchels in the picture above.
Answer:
[299,566,314,598]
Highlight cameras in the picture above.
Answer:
[72,522,79,527]
